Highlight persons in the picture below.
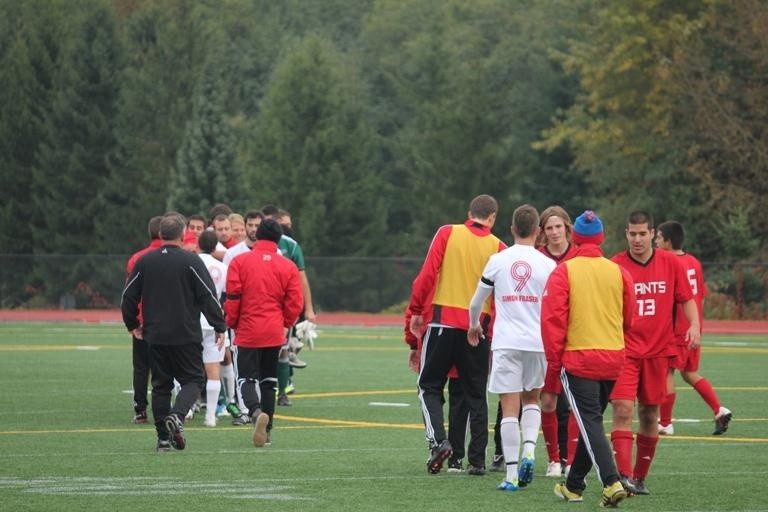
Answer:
[224,219,303,447]
[126,202,315,426]
[541,209,636,507]
[655,222,732,435]
[121,215,226,451]
[609,211,700,497]
[406,195,582,490]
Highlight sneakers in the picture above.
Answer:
[425,439,650,508]
[131,351,307,453]
[712,407,732,434]
[658,424,674,435]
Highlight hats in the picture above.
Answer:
[570,210,604,244]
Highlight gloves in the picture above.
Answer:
[295,320,318,352]
